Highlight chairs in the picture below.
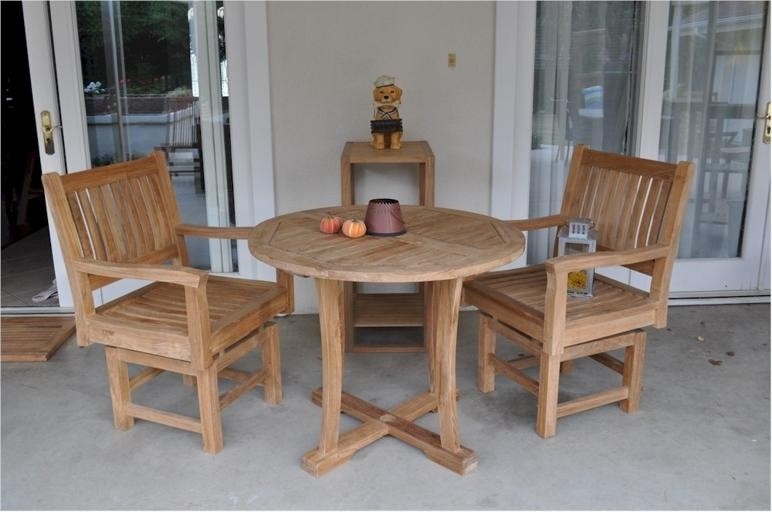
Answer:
[41,151,294,453]
[155,98,203,193]
[463,144,696,438]
[550,98,593,168]
[720,145,751,197]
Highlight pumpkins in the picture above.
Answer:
[342,217,367,238]
[320,212,343,234]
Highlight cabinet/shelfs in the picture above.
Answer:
[340,141,436,353]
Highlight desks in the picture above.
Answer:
[249,205,525,476]
[667,103,756,259]
